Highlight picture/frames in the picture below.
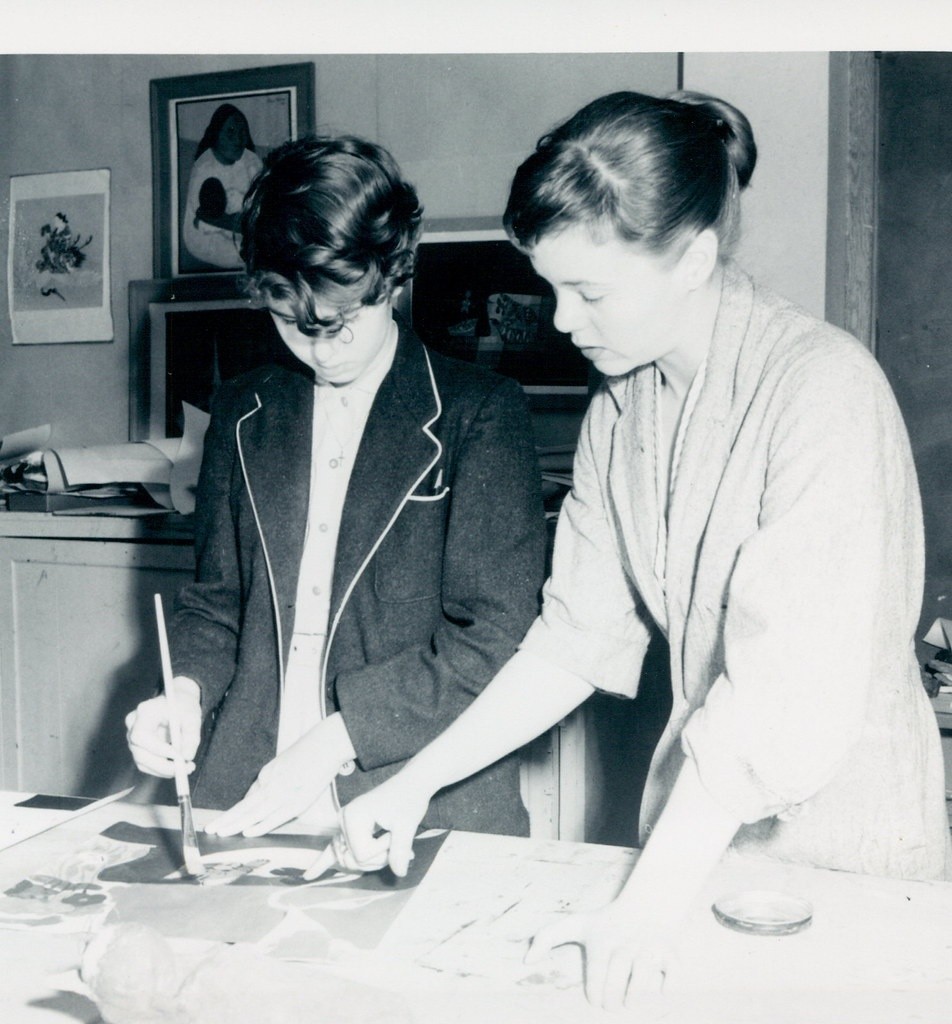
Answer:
[149,61,317,280]
[396,215,605,413]
[126,270,288,441]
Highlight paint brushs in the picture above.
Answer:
[152,589,207,877]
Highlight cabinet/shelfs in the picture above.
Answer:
[0,535,193,799]
[516,693,617,843]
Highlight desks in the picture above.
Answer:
[0,789,952,1024]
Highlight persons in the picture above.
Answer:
[303,91,952,1010]
[125,135,546,837]
